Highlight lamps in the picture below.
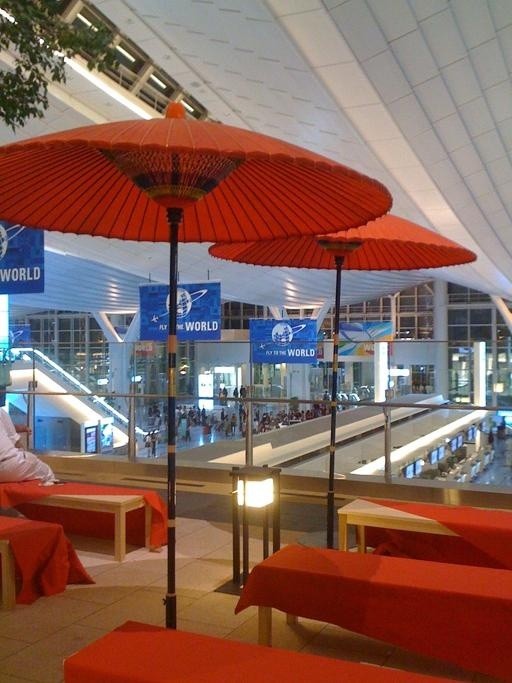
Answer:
[229,466,282,587]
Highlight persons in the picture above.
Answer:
[414,370,421,391]
[143,382,346,459]
[488,431,494,444]
[420,368,428,392]
[0,408,56,482]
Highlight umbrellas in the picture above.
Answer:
[206,212,481,547]
[0,103,393,630]
